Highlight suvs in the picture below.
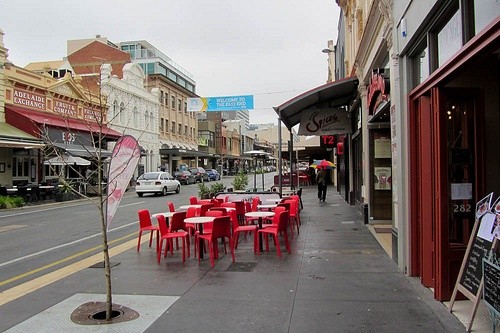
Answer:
[189,167,210,183]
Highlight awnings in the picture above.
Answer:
[272,75,360,199]
[44,143,208,166]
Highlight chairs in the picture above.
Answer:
[156,195,300,267]
[137,210,159,252]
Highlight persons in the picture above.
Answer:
[316,166,329,203]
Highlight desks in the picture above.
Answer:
[184,217,215,258]
[179,205,201,214]
[152,212,179,228]
[247,198,282,220]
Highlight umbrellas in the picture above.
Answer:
[309,159,336,170]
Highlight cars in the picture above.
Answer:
[135,171,182,197]
[206,169,221,181]
[172,171,197,185]
[250,165,288,173]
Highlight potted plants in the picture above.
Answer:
[198,183,225,199]
